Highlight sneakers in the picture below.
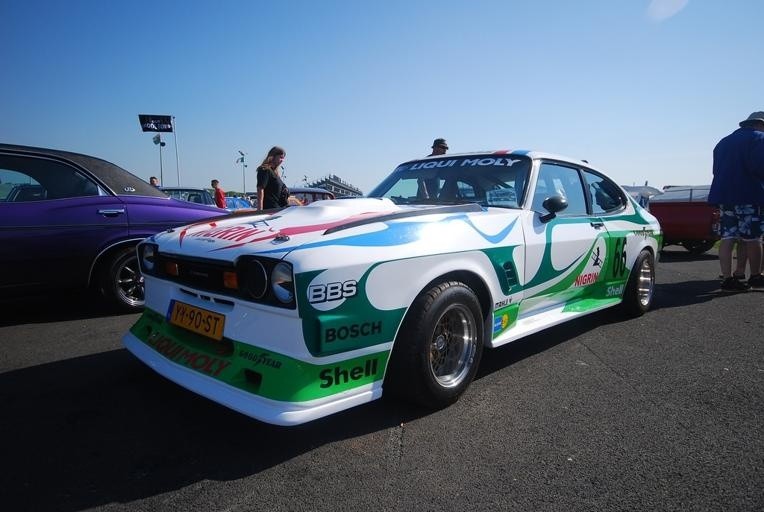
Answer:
[719,271,764,292]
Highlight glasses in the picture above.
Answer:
[440,146,450,150]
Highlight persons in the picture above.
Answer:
[255,147,291,211]
[705,108,764,291]
[718,236,747,280]
[151,178,162,192]
[211,179,230,210]
[415,138,450,200]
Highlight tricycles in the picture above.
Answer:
[620,185,725,254]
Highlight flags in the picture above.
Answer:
[138,114,174,133]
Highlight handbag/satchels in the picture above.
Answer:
[278,183,288,207]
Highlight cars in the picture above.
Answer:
[121,148,665,427]
[156,185,335,209]
[0,142,235,312]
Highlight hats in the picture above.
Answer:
[431,139,448,148]
[739,111,764,127]
